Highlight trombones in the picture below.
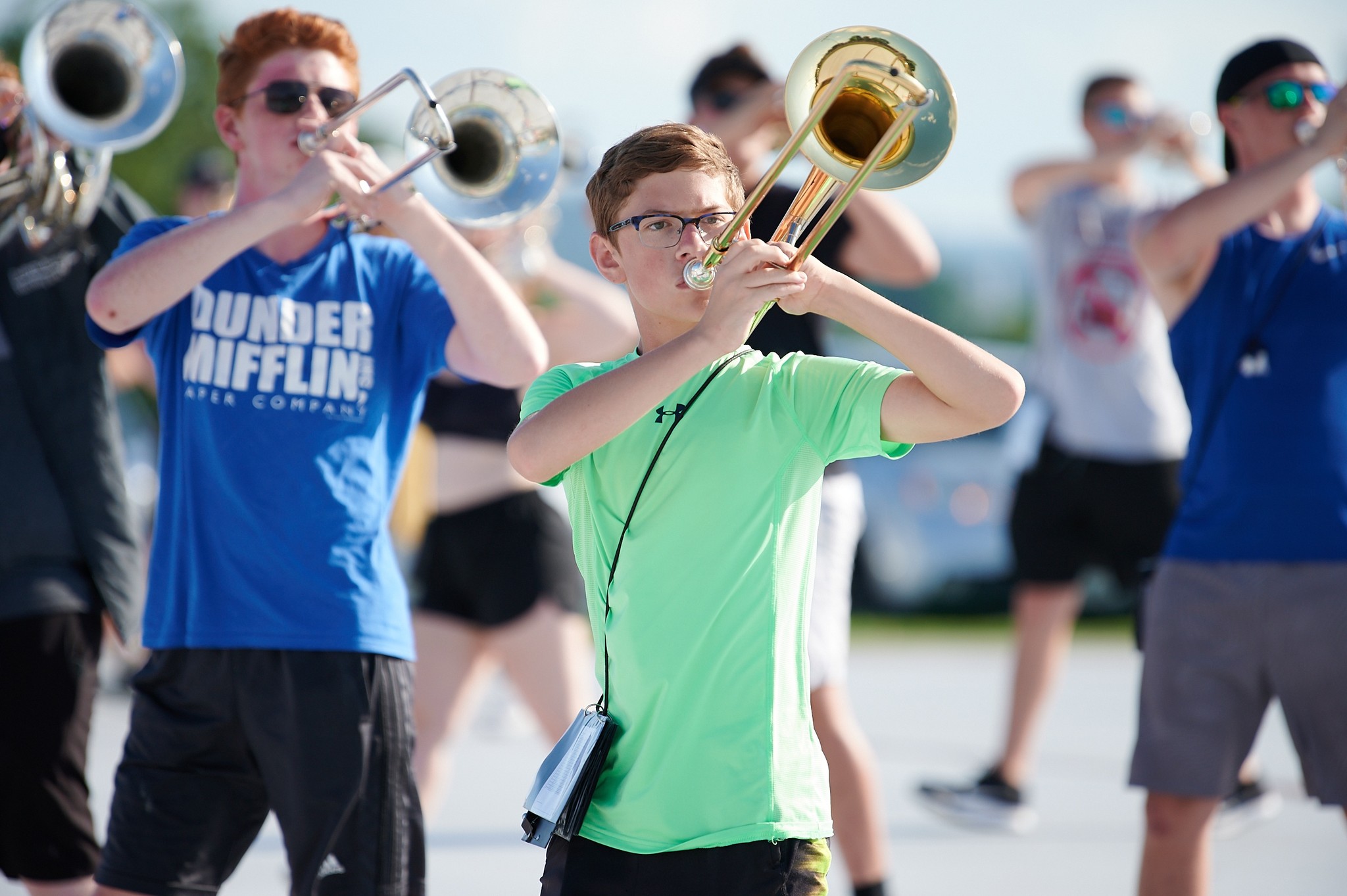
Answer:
[294,57,564,234]
[683,22,960,330]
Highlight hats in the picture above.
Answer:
[691,46,772,102]
[1216,40,1323,176]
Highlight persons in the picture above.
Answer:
[1,60,157,895]
[99,38,1281,895]
[1127,36,1347,896]
[503,125,1027,896]
[86,6,548,896]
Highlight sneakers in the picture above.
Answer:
[1207,775,1272,821]
[922,769,1029,835]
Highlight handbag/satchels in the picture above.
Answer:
[1130,522,1163,649]
[521,708,618,849]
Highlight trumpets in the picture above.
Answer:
[1,0,188,255]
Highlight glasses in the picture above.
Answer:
[1249,79,1339,110]
[230,79,355,118]
[607,212,745,249]
